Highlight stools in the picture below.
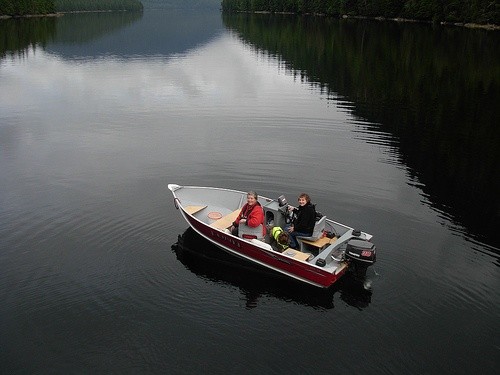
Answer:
[299,237,331,254]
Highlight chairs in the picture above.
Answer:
[238,219,263,239]
[297,216,326,241]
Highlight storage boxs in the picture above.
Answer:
[283,248,312,263]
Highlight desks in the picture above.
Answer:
[262,200,288,229]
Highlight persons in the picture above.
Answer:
[231,191,267,237]
[284,193,317,249]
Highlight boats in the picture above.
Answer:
[167,183,377,290]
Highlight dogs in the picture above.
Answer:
[269,226,291,247]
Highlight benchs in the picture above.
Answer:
[185,205,242,232]
